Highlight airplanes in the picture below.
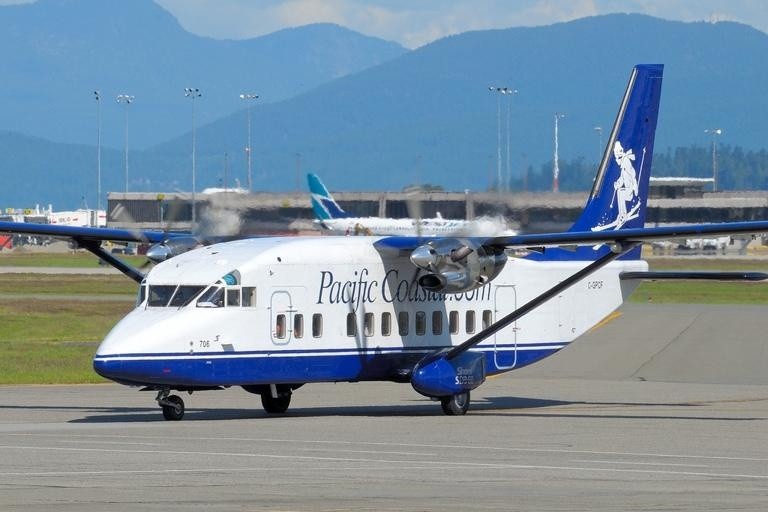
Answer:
[0,64,767,421]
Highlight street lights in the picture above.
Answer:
[704,130,721,191]
[184,87,202,234]
[94,91,103,209]
[501,90,517,193]
[489,87,508,194]
[117,93,135,191]
[240,95,259,190]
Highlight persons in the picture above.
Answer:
[611,141,639,222]
